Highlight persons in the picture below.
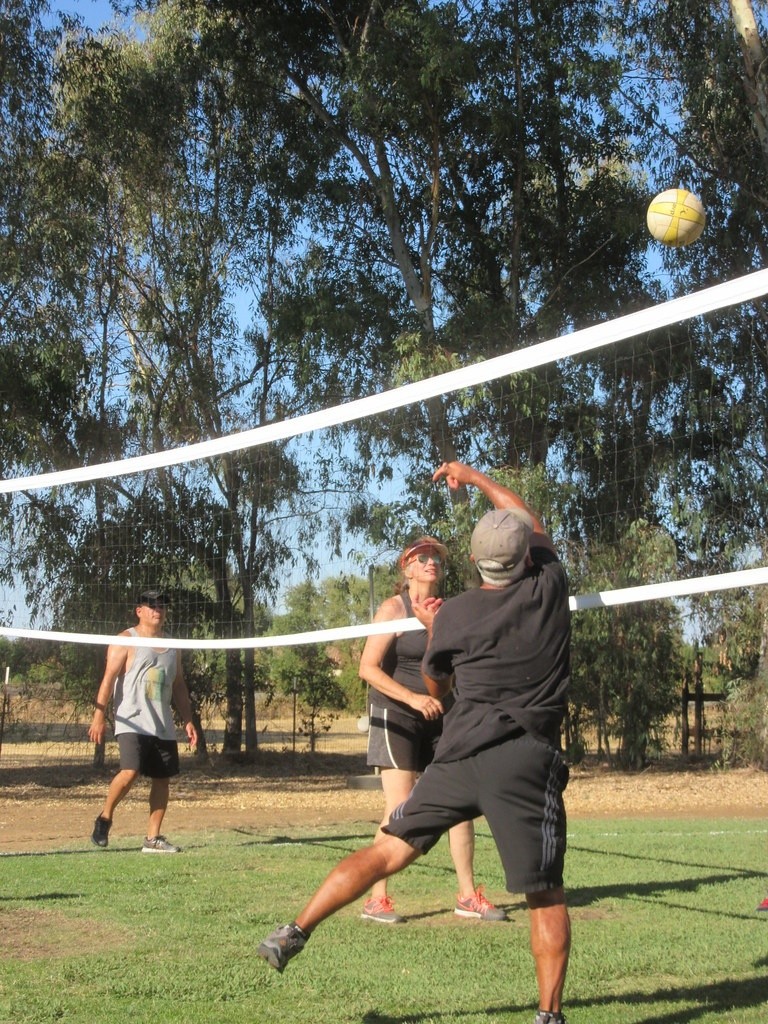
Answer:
[88,590,197,853]
[257,462,571,1024]
[359,538,507,924]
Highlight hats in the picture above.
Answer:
[470,507,533,572]
[137,591,171,600]
[400,536,448,560]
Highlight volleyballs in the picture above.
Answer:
[645,187,707,249]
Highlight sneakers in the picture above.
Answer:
[453,884,507,921]
[533,1009,566,1024]
[257,924,305,974]
[89,810,113,848]
[360,896,407,923]
[141,835,182,853]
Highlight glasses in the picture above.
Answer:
[405,555,442,567]
[148,602,164,608]
